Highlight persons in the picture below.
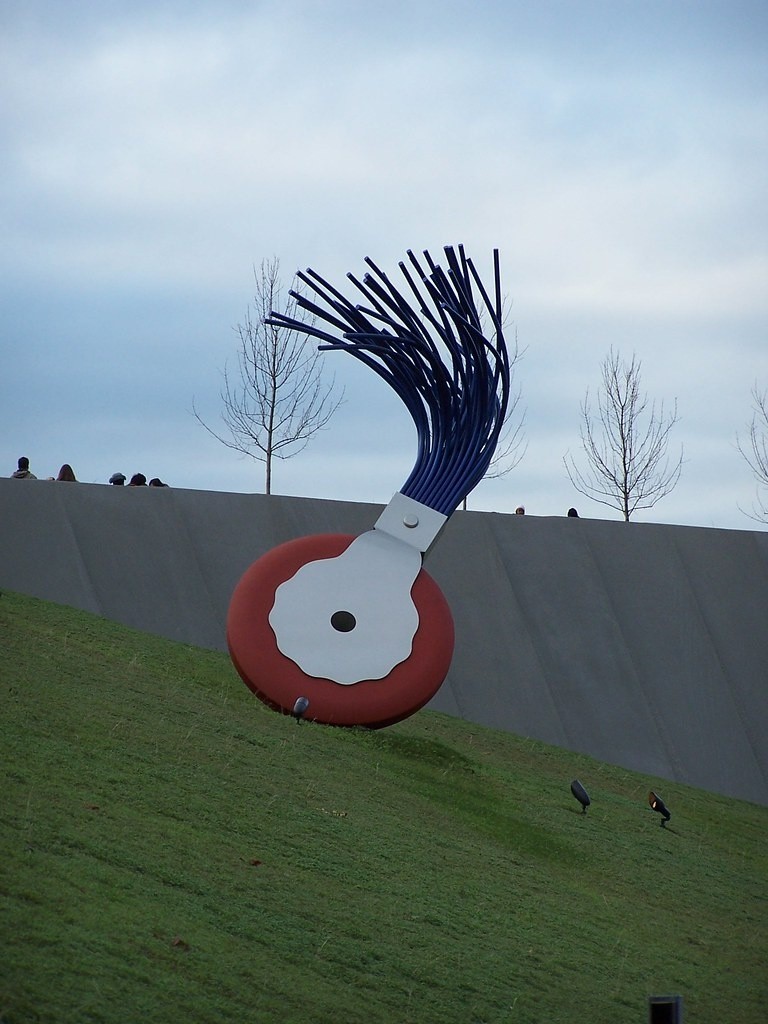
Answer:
[126,472,147,486]
[568,507,578,517]
[9,457,37,479]
[109,472,126,485]
[515,504,525,515]
[48,464,76,481]
[149,478,168,487]
